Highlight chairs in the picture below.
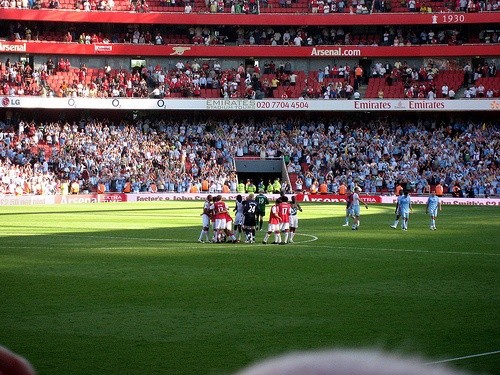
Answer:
[0,0,500,195]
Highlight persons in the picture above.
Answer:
[0,0,500,199]
[255,190,269,231]
[210,195,227,243]
[342,185,354,227]
[233,195,244,242]
[288,196,302,243]
[201,196,238,243]
[262,200,281,244]
[399,192,413,231]
[351,187,368,230]
[198,195,212,243]
[424,190,442,231]
[243,194,259,244]
[390,190,406,228]
[279,197,290,244]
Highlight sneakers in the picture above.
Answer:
[342,222,437,231]
[196,227,295,245]
